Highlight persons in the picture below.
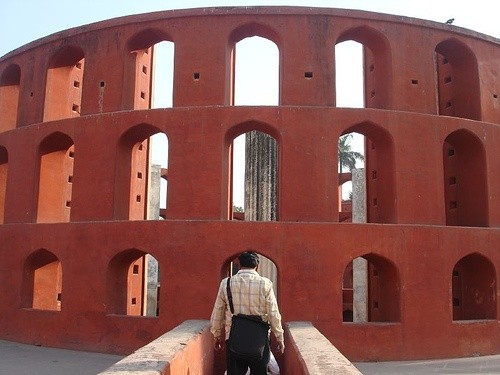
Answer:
[210,250,287,375]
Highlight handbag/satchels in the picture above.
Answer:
[226,313,272,360]
